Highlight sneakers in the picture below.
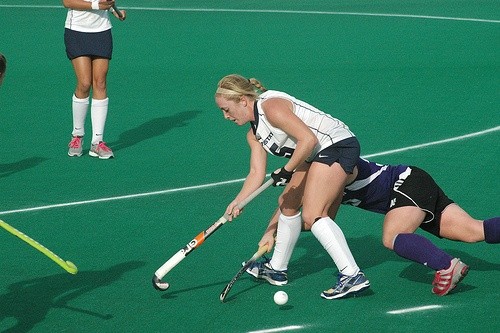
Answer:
[432,258,470,296]
[321,271,371,299]
[68,136,84,157]
[241,255,288,286]
[88,141,114,159]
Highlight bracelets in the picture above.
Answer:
[92,0,99,10]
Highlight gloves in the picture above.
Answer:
[270,167,294,187]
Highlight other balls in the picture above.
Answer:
[273,290,288,305]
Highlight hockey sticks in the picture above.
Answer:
[219,243,269,302]
[0,219,78,275]
[107,0,125,20]
[151,177,275,291]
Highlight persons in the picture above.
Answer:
[63,0,126,158]
[214,74,370,300]
[258,156,500,296]
